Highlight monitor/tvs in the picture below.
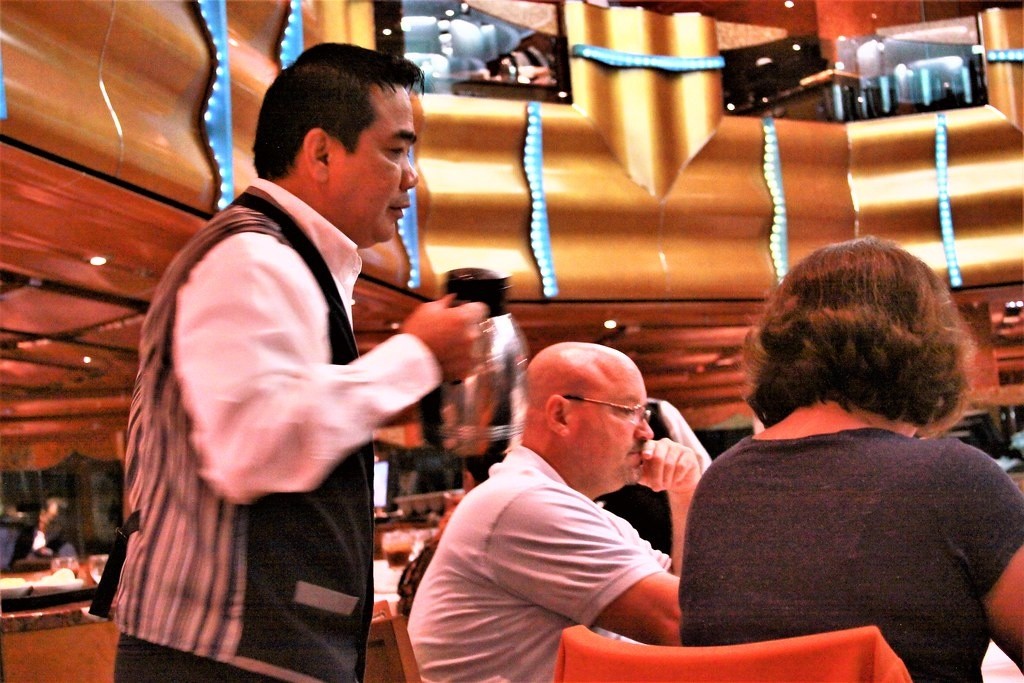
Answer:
[367,0,573,104]
[835,11,990,123]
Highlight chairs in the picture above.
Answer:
[554,624,913,683]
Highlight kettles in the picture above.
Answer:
[419,266,529,458]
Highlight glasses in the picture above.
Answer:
[561,394,652,428]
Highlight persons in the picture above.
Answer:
[89,42,492,682]
[407,340,703,683]
[397,271,527,622]
[678,235,1024,682]
[1,497,80,574]
